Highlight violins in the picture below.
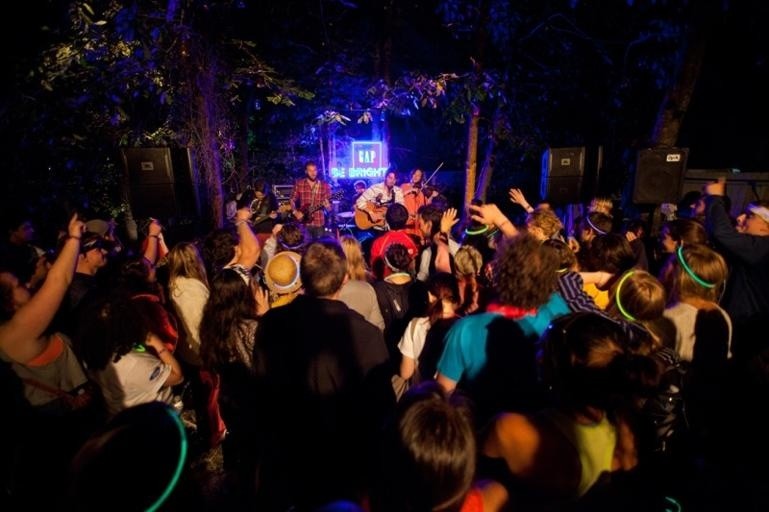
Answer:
[414,181,450,202]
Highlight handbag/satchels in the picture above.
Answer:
[62,385,96,412]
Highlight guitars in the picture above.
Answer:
[289,191,343,223]
[355,201,415,231]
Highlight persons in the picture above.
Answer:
[2,162,768,511]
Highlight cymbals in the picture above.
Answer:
[337,212,357,217]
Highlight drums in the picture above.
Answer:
[338,225,360,239]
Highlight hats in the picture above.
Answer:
[79,231,118,254]
[264,251,303,302]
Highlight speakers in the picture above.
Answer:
[540,146,585,175]
[631,147,688,204]
[121,146,192,184]
[537,176,584,215]
[125,181,196,246]
